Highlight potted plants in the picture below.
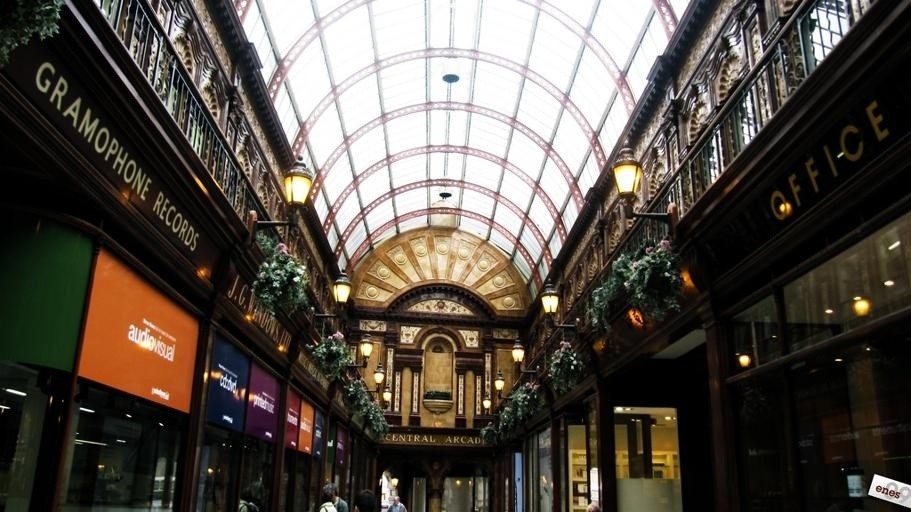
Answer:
[248,243,391,441]
[479,238,682,445]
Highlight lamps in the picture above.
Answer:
[248,153,312,238]
[610,136,678,221]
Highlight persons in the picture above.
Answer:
[237,484,259,512]
[354,489,378,512]
[387,496,407,512]
[586,502,602,512]
[323,484,348,512]
[318,493,338,512]
